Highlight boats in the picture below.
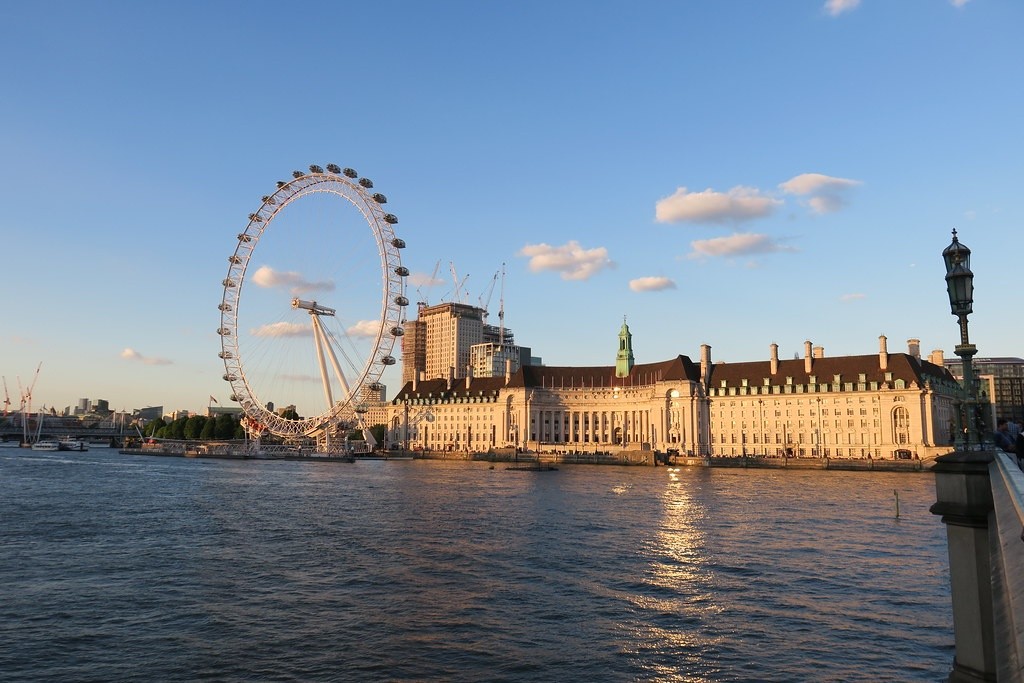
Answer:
[60,435,89,452]
[31,440,61,451]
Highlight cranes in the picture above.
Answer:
[1,376,12,417]
[25,360,44,430]
[416,259,506,343]
[17,376,27,431]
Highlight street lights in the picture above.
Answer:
[941,228,983,452]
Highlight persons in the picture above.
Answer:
[1016,422,1024,469]
[993,419,1015,453]
[1006,417,1018,440]
